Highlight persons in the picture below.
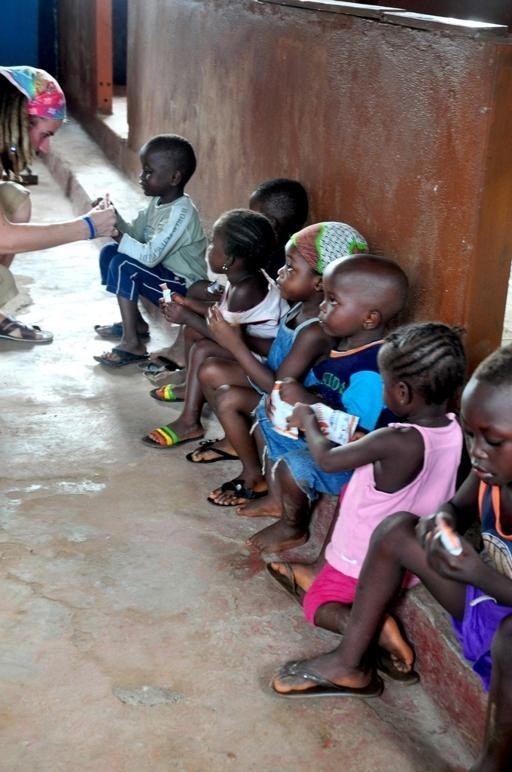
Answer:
[265,321,472,688]
[0,65,118,343]
[137,177,309,387]
[268,346,512,772]
[236,253,409,554]
[140,208,291,449]
[186,222,370,509]
[91,134,208,367]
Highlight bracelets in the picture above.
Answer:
[82,216,94,239]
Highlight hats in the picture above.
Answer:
[290,221,372,275]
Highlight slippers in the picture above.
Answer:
[184,437,242,464]
[208,471,272,511]
[92,347,152,368]
[93,322,151,340]
[271,640,420,699]
[141,424,206,450]
[136,354,182,372]
[148,382,187,404]
[0,315,56,343]
[261,556,311,610]
